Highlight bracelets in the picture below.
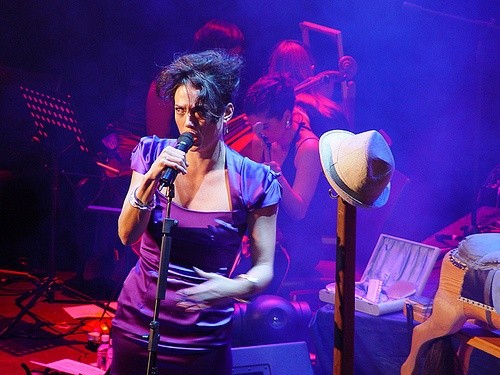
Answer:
[128,185,156,211]
[232,273,257,304]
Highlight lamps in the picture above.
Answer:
[229,297,312,345]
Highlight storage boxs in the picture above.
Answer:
[318,233,440,316]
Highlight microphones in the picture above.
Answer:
[159,132,194,187]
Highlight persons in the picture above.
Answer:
[245,76,331,296]
[147,21,244,141]
[111,53,285,375]
[270,38,347,214]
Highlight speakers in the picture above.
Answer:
[81,206,122,255]
[231,340,314,374]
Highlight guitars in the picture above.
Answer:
[226,54,358,153]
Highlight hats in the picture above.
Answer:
[319,128,396,212]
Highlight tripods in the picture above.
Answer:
[0,85,120,344]
